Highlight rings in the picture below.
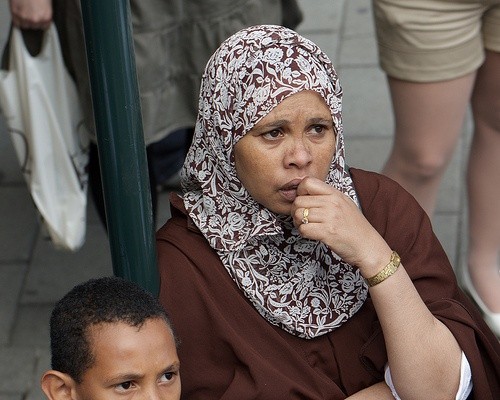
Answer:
[301,208,308,225]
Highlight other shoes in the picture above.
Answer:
[461,259,500,340]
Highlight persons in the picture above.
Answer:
[153,24,500,400]
[371,0,500,333]
[1,0,306,238]
[38,276,181,400]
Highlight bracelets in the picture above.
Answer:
[366,249,401,288]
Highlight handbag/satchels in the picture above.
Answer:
[0,21,91,252]
